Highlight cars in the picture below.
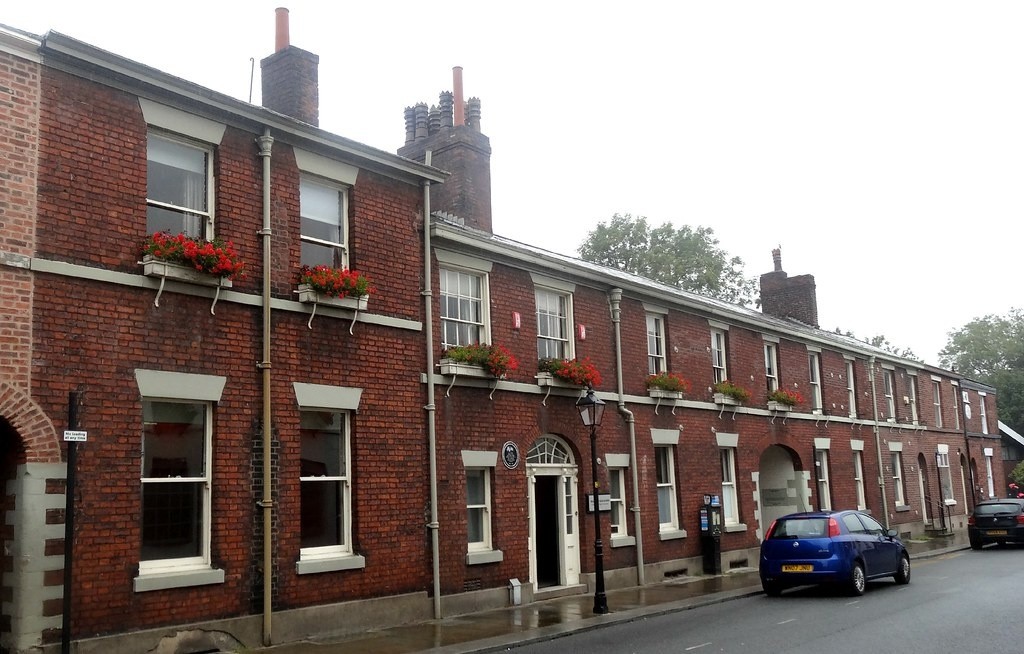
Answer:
[760,509,910,597]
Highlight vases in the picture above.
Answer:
[299,284,368,312]
[646,387,683,399]
[536,372,584,390]
[442,359,507,380]
[714,393,741,407]
[144,256,233,290]
[768,400,792,412]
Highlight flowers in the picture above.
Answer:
[644,372,691,392]
[538,357,602,389]
[768,388,803,406]
[446,341,520,379]
[713,380,752,401]
[298,265,378,299]
[141,230,244,280]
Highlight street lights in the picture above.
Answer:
[576,382,609,615]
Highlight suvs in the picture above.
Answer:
[967,498,1024,550]
